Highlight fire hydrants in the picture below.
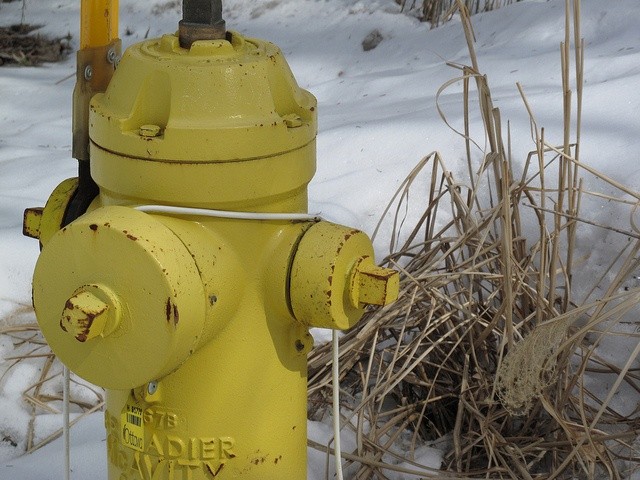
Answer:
[23,0,399,480]
